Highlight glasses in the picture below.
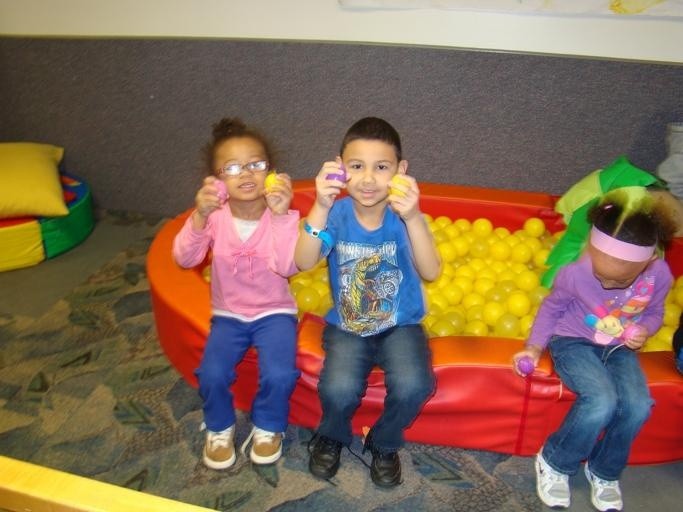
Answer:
[214,158,270,176]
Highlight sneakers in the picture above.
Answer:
[202,423,237,469]
[240,426,285,465]
[535,447,571,508]
[584,460,623,512]
[365,432,401,488]
[307,431,342,487]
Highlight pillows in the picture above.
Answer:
[0,141,69,217]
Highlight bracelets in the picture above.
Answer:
[303,221,332,248]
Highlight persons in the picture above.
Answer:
[512,200,674,512]
[293,116,441,489]
[171,115,302,471]
